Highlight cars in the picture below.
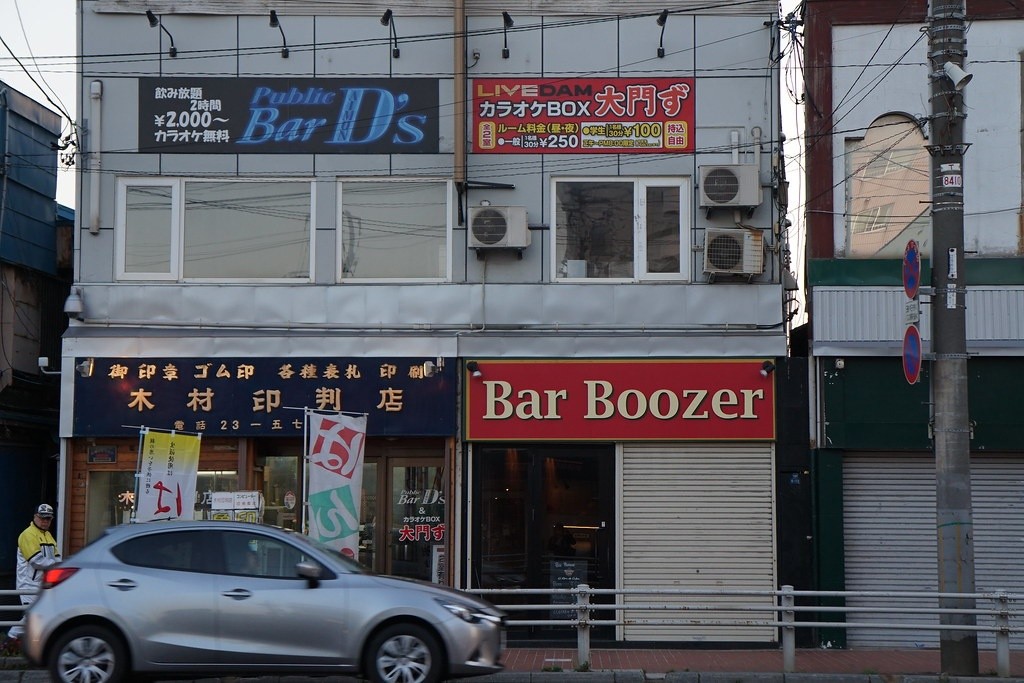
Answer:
[23,520,506,683]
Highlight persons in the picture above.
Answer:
[2,503,62,649]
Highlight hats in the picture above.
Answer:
[35,502,54,519]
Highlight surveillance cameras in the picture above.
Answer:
[64,286,84,319]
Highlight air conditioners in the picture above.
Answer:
[701,225,766,284]
[464,201,533,263]
[694,159,764,220]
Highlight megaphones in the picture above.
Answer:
[944,61,973,92]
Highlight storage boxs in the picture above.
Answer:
[207,490,266,524]
